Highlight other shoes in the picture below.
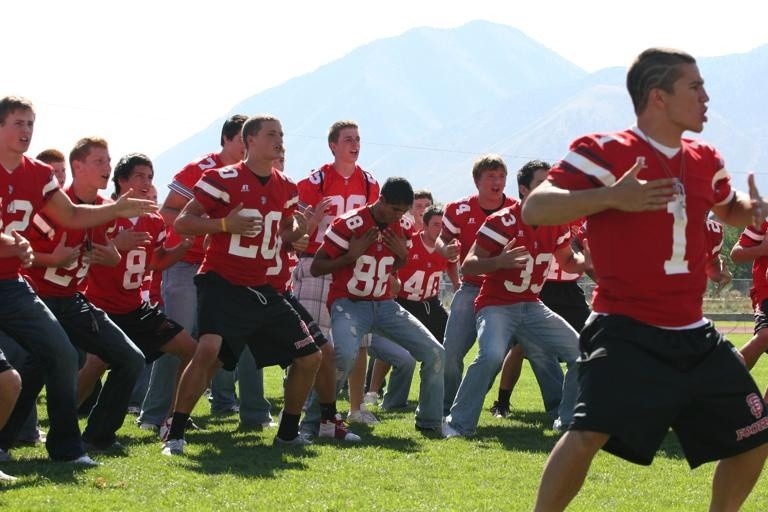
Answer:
[337,385,384,405]
[209,401,240,412]
[81,433,127,457]
[16,426,47,443]
[1,472,19,482]
[2,451,16,462]
[205,388,214,399]
[50,455,103,468]
[241,414,278,429]
[127,405,201,458]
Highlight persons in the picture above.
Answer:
[1,96,597,478]
[730,220,768,405]
[518,46,768,512]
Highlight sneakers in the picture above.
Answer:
[346,403,379,424]
[319,413,361,441]
[441,417,476,439]
[415,420,447,443]
[489,400,513,420]
[551,416,567,432]
[298,422,318,443]
[273,434,312,446]
